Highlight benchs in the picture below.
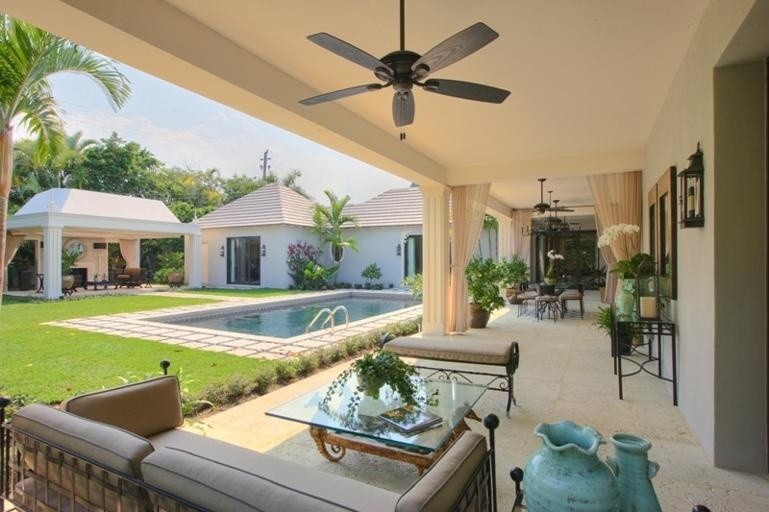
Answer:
[380,331,519,411]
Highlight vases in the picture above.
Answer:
[523,421,662,512]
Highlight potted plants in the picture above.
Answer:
[588,253,658,357]
[465,254,533,328]
[62,247,83,289]
[158,250,184,282]
[321,349,439,420]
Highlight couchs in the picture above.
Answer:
[0,360,499,512]
[120,267,146,287]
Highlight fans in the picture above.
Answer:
[512,179,589,243]
[298,0,511,141]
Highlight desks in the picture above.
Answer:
[613,310,677,405]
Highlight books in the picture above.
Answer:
[376,404,443,433]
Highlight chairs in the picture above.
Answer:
[515,282,584,321]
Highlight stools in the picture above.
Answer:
[114,274,134,289]
